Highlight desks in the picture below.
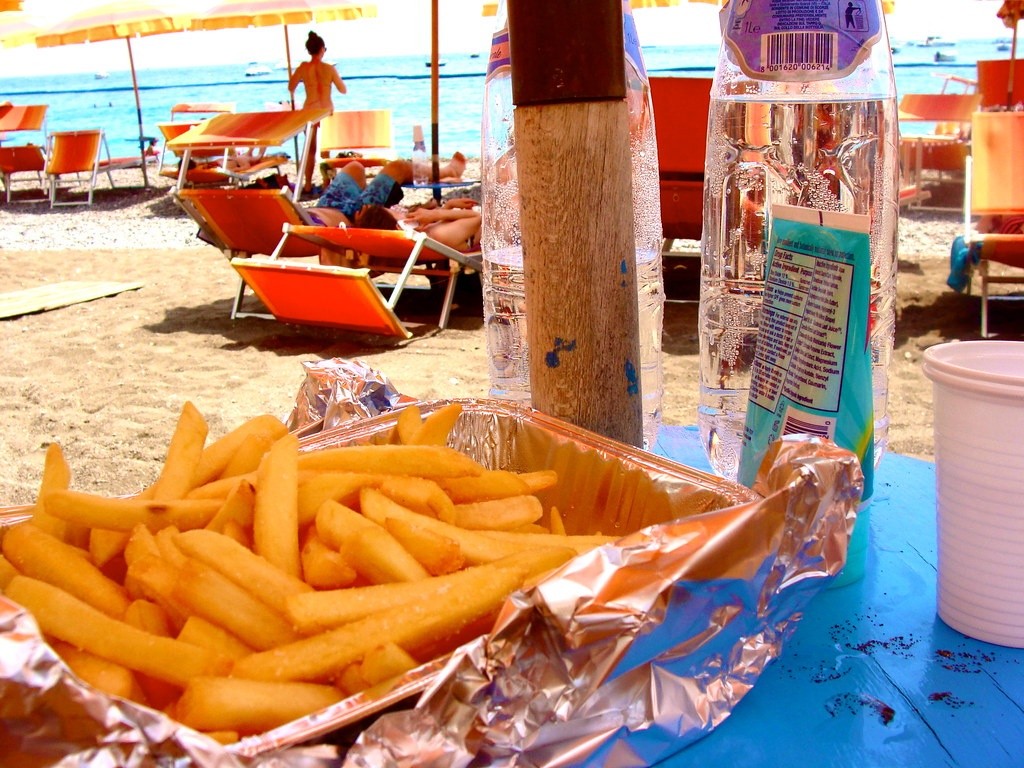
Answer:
[402,181,481,198]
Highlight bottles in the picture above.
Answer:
[481,0,666,454]
[413,126,429,186]
[698,0,899,485]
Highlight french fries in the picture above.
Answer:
[0,403,625,744]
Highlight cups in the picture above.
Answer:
[922,341,1024,649]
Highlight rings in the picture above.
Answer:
[417,218,418,224]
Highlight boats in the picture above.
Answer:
[425,59,449,67]
[245,58,336,76]
[891,36,1010,64]
[95,71,110,79]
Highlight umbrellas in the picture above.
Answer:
[0,0,43,50]
[190,0,379,176]
[35,0,190,191]
[481,0,728,16]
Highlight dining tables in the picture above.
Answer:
[579,424,1024,768]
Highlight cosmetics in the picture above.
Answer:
[738,203,873,590]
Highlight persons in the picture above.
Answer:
[145,139,160,156]
[178,145,267,172]
[303,151,465,225]
[363,196,483,253]
[288,30,346,193]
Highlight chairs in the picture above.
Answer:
[0,100,482,347]
[896,76,1023,338]
